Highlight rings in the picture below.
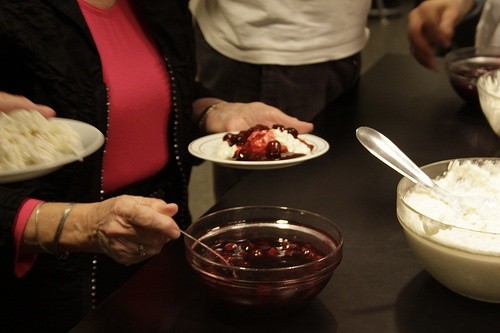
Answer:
[138,243,144,257]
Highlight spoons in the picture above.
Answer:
[179,229,258,283]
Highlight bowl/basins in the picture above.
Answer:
[444,46,500,109]
[477,69,500,136]
[184,205,343,321]
[396,157,499,303]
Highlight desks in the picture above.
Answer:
[76,52,500,333]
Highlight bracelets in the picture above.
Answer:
[198,101,222,127]
[34,201,46,244]
[54,202,74,258]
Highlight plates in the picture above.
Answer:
[188,131,329,169]
[0,117,105,184]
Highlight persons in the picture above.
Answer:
[0,0,314,297]
[186,0,373,202]
[405,0,500,71]
